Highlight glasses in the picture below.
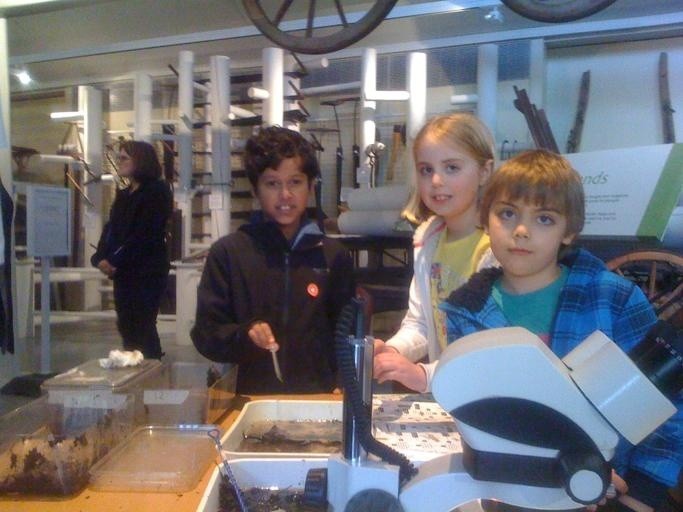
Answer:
[118,154,133,162]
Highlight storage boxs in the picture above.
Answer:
[1,357,239,500]
[196,400,343,512]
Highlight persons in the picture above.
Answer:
[424,148,683,512]
[88,139,174,363]
[358,111,508,397]
[188,124,360,396]
